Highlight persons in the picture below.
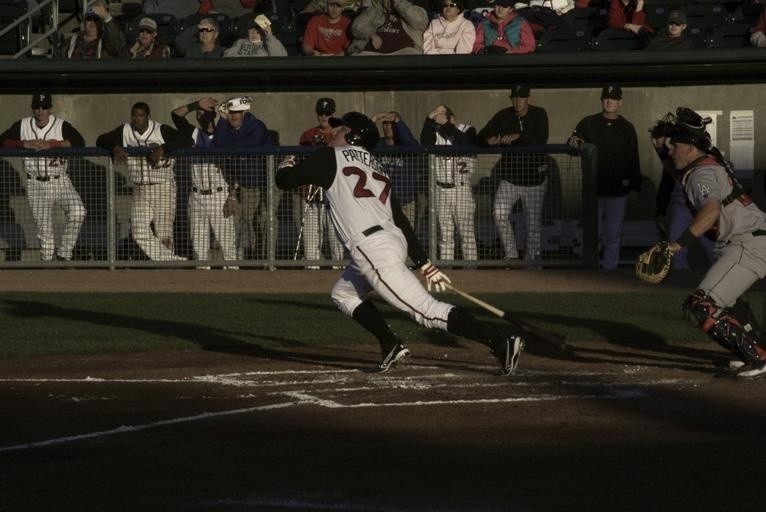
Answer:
[170,98,239,271]
[567,83,643,272]
[96,102,189,261]
[210,97,267,272]
[275,110,528,376]
[475,83,551,270]
[422,1,536,54]
[651,120,711,273]
[371,111,419,232]
[635,120,766,381]
[420,104,476,270]
[65,3,126,59]
[295,0,428,56]
[0,92,88,261]
[296,97,345,269]
[125,15,170,57]
[542,2,765,52]
[172,1,288,57]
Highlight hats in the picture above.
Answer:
[315,98,335,114]
[508,83,531,99]
[138,17,157,31]
[601,85,622,102]
[31,92,52,108]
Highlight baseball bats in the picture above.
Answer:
[444,283,566,351]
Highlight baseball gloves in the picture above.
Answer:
[635,241,675,284]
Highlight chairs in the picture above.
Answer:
[115,2,758,56]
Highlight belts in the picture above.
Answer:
[436,181,464,189]
[363,225,384,237]
[193,186,222,195]
[25,173,61,181]
[751,228,765,235]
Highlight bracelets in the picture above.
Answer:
[187,100,199,112]
[228,197,236,201]
[394,114,399,123]
[5,139,23,148]
[675,229,697,248]
[48,141,64,148]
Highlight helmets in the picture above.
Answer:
[328,111,379,153]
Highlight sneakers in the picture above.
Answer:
[729,354,766,378]
[378,343,410,375]
[498,335,526,378]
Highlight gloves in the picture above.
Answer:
[277,155,295,169]
[419,259,450,293]
